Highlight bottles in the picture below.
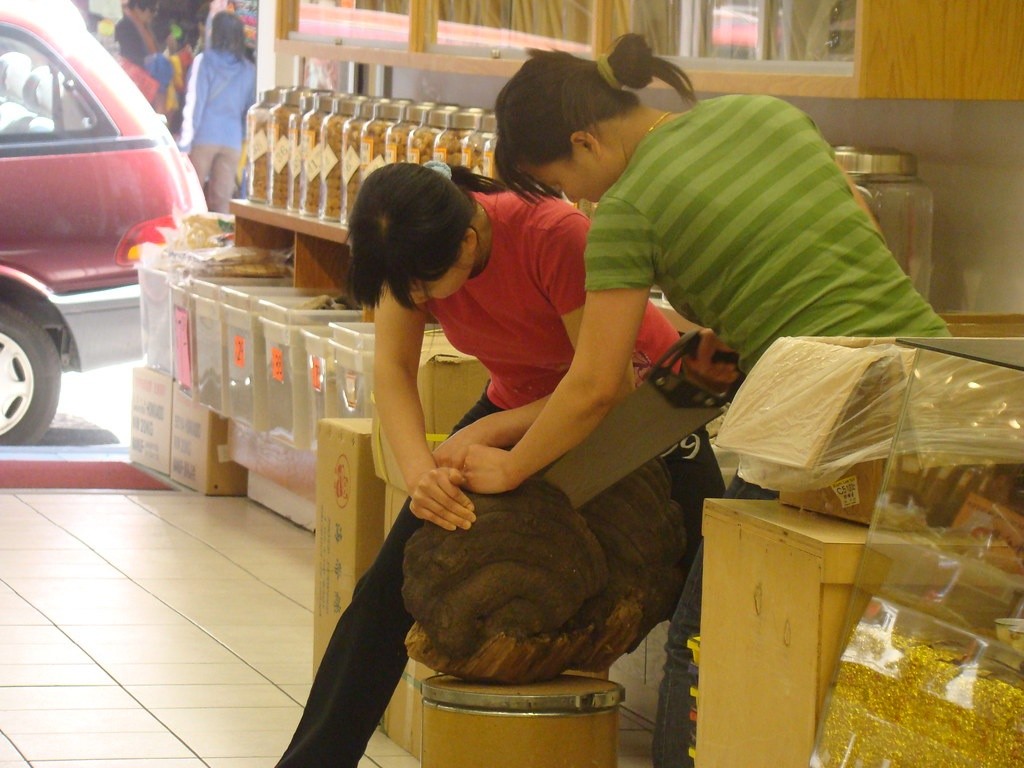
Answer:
[834,144,933,312]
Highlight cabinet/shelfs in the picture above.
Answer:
[222,0,1024,101]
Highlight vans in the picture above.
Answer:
[0,0,208,446]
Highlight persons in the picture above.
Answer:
[451,32,955,768]
[274,163,727,768]
[73,0,257,213]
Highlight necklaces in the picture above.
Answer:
[644,110,673,133]
[477,203,489,271]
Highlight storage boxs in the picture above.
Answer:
[128,261,1024,761]
